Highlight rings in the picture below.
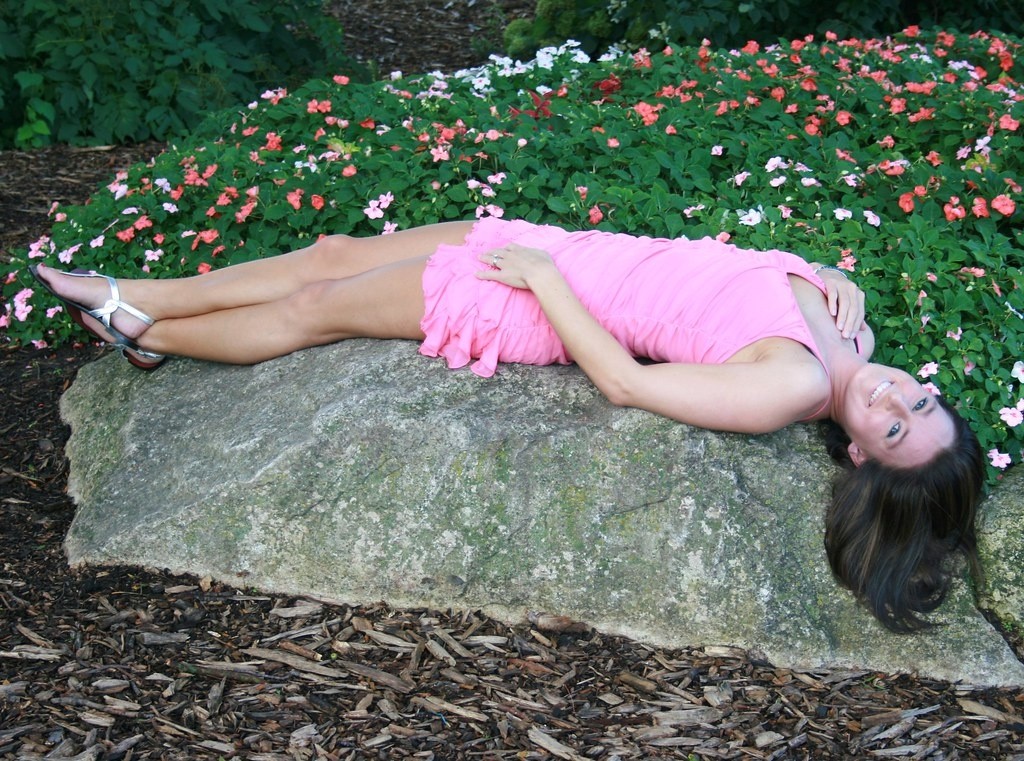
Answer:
[493,257,501,264]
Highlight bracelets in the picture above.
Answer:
[815,265,847,276]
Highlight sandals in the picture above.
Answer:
[29,262,156,351]
[66,269,165,369]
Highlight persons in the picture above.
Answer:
[33,218,985,631]
